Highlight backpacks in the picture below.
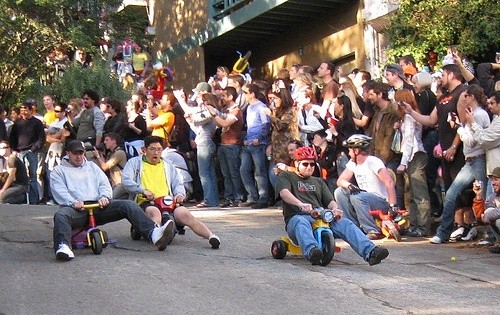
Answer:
[120,142,139,161]
[160,114,190,147]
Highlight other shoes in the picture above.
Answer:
[364,226,500,252]
[197,198,268,209]
[55,221,220,260]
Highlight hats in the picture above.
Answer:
[67,140,85,152]
[487,167,500,178]
[100,97,111,105]
[192,82,211,93]
[386,65,405,79]
[26,99,36,104]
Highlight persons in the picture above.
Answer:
[48,37,148,80]
[332,134,397,240]
[0,71,222,208]
[49,140,173,259]
[122,136,221,249]
[275,147,390,266]
[473,167,500,253]
[206,50,500,243]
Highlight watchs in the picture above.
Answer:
[390,204,397,208]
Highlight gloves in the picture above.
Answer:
[348,184,360,195]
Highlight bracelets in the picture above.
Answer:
[451,145,456,149]
[213,113,218,118]
[97,156,100,158]
[334,132,338,136]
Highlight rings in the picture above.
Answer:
[450,157,452,158]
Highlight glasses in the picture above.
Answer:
[490,102,497,105]
[301,162,315,168]
[20,106,27,109]
[464,94,470,98]
[243,91,248,95]
[54,109,61,112]
[0,147,9,149]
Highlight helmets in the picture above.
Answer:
[292,147,318,160]
[346,134,372,152]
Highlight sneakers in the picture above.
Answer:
[308,247,323,265]
[368,246,389,265]
